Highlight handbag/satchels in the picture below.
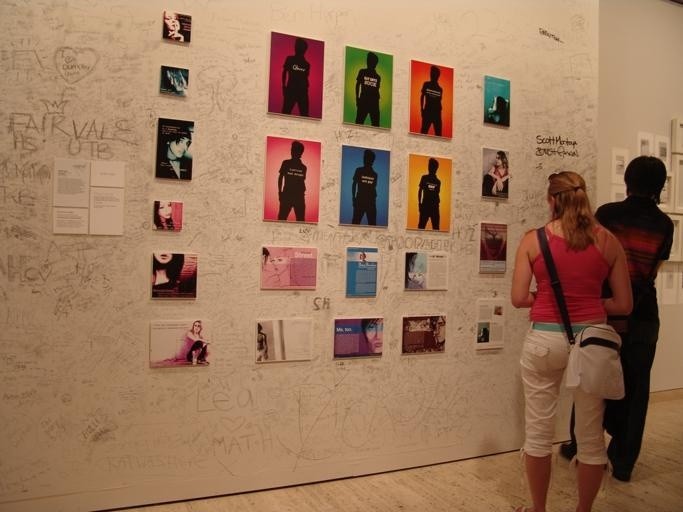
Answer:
[566,325,627,402]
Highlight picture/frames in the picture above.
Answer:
[610,117,682,262]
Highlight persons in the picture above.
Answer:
[362,318,382,356]
[156,201,176,227]
[511,171,634,512]
[485,150,509,195]
[355,52,381,127]
[350,150,378,225]
[275,140,307,221]
[489,96,508,126]
[156,253,183,290]
[414,158,441,230]
[164,13,189,42]
[185,320,207,366]
[282,37,312,116]
[358,253,367,266]
[156,128,192,179]
[256,324,268,361]
[164,70,188,95]
[560,156,674,482]
[419,66,443,137]
[427,317,445,352]
